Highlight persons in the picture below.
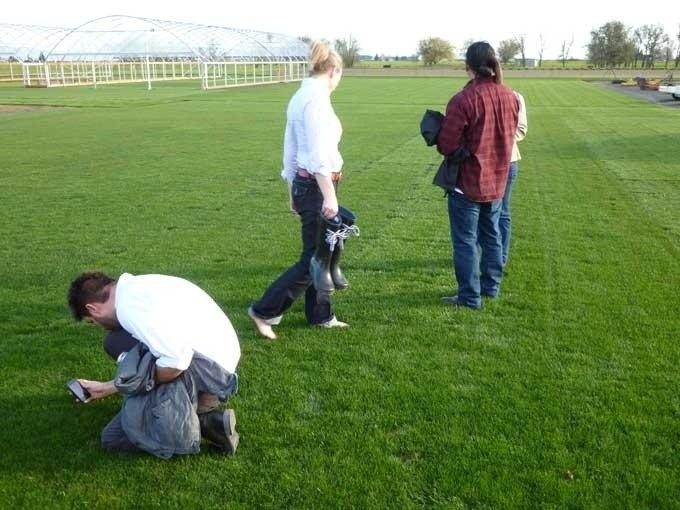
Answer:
[67,271,243,460]
[246,38,351,342]
[490,56,529,266]
[435,39,520,309]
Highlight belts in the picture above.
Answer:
[297,169,343,181]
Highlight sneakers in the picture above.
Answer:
[440,261,508,311]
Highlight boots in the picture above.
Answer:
[331,205,356,290]
[199,408,240,457]
[309,208,336,296]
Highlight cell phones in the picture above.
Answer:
[67,379,92,403]
